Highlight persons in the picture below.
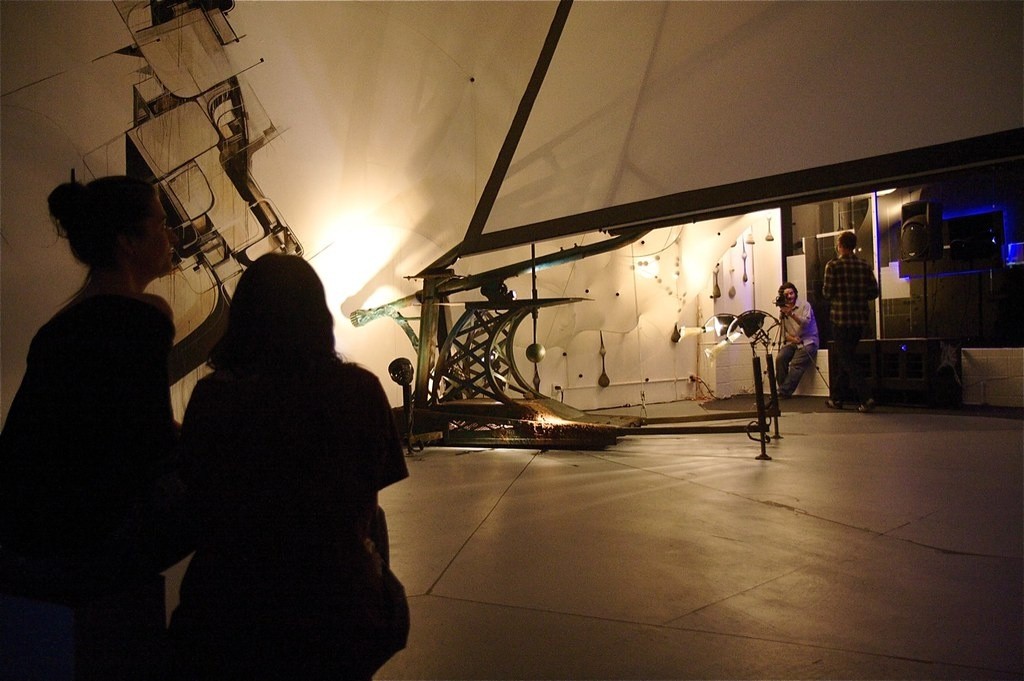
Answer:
[1,174,412,681]
[823,231,880,412]
[774,282,819,399]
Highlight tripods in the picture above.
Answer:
[762,313,830,394]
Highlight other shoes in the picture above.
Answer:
[770,392,790,399]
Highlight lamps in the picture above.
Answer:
[679,310,780,358]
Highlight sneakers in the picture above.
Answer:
[826,400,843,408]
[857,399,874,412]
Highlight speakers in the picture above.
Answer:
[900,200,942,261]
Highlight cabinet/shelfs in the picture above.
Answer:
[827,337,962,408]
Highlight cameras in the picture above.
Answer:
[775,293,788,306]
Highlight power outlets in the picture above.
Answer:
[687,371,696,383]
[552,382,561,392]
